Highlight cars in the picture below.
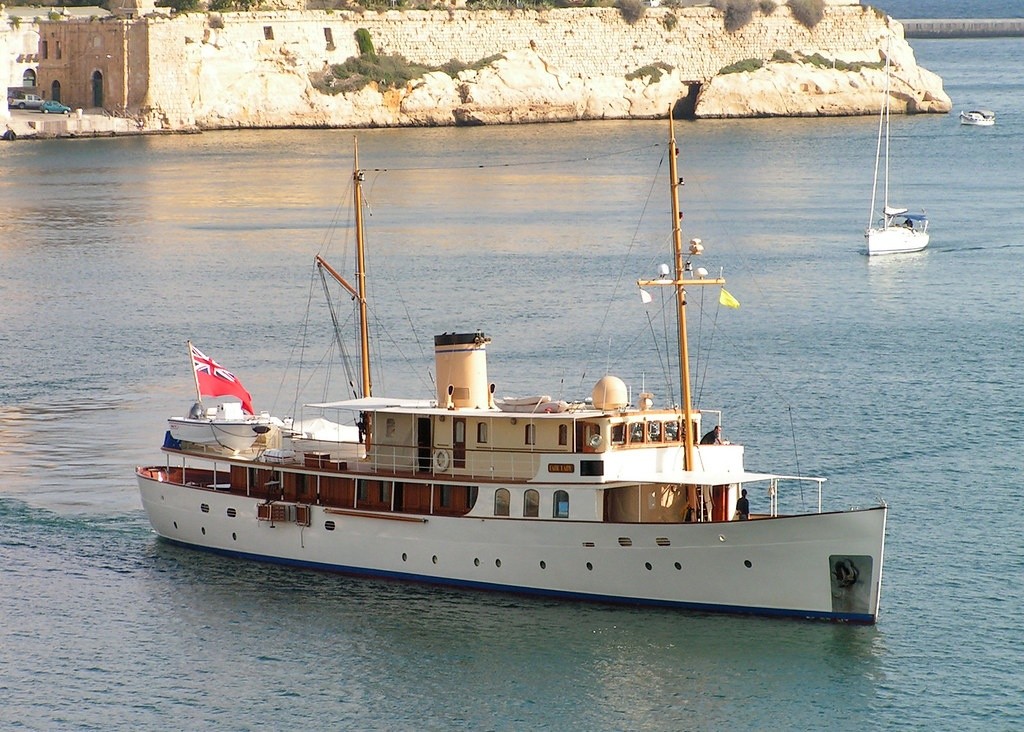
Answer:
[41,99,71,115]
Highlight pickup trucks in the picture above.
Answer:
[8,93,46,110]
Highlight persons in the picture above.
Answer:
[736,489,749,520]
[700,425,722,445]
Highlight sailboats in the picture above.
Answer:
[864,30,931,257]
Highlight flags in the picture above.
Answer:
[191,345,254,415]
[640,289,652,304]
[718,288,740,309]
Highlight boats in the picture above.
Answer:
[165,339,272,452]
[134,101,890,627]
[959,108,996,127]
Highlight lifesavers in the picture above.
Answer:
[433,449,450,472]
